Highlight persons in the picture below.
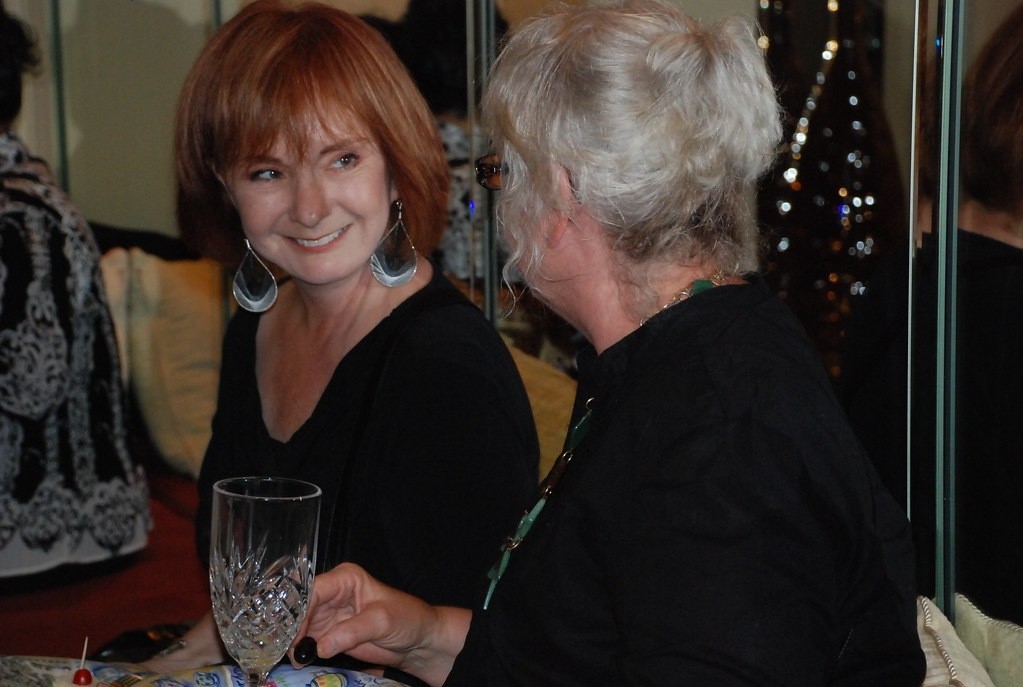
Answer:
[137,0,1023,687]
[0,0,155,577]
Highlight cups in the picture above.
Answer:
[209,477,321,687]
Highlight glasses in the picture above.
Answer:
[474,152,580,204]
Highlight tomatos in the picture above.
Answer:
[72,668,93,685]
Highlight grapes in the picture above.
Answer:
[294,636,318,664]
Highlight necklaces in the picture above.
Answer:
[481,265,725,612]
